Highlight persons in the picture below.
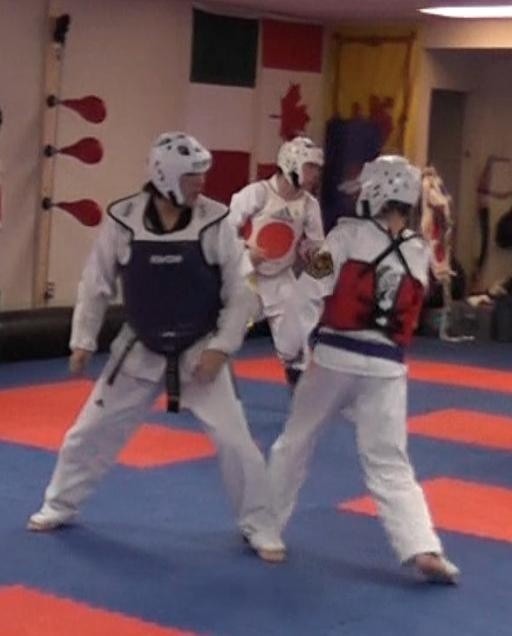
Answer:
[26,131,288,564]
[261,155,462,586]
[219,136,335,398]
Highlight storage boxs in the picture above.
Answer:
[449,296,498,343]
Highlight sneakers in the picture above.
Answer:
[240,520,286,562]
[407,551,458,582]
[28,505,67,529]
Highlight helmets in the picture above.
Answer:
[147,130,212,203]
[278,136,325,186]
[357,156,421,214]
